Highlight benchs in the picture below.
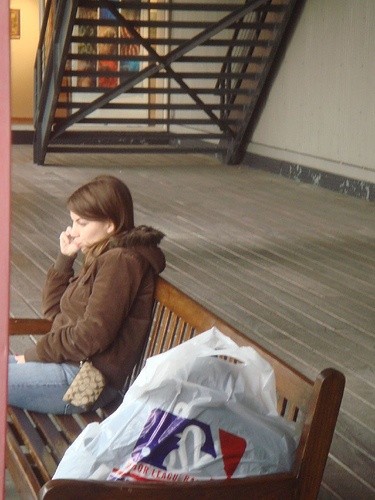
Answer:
[6,268,347,500]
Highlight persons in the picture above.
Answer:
[9,174,168,415]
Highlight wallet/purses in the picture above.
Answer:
[62,360,106,415]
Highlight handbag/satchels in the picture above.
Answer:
[50,327,301,481]
